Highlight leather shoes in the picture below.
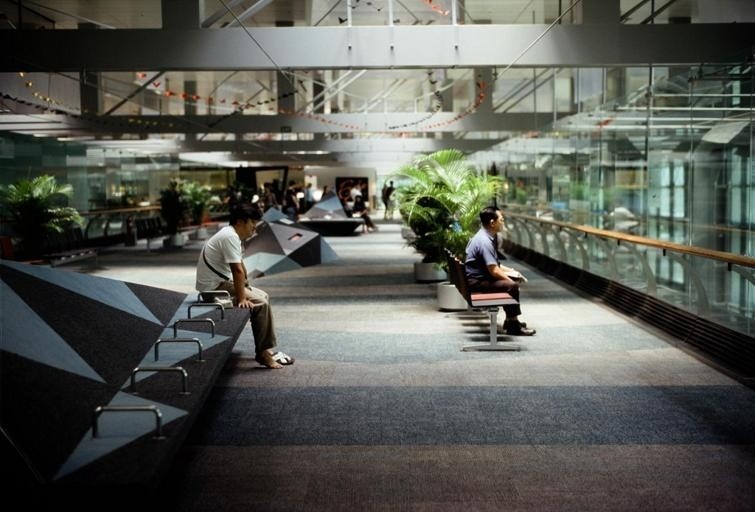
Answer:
[503,320,535,335]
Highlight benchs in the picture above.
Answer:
[441,247,526,352]
[52,288,252,509]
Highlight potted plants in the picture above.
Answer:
[390,148,506,310]
[157,188,208,247]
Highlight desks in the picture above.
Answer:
[79,204,161,240]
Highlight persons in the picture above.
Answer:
[220,177,397,236]
[194,201,284,370]
[463,205,537,336]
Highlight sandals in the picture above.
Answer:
[272,351,295,364]
